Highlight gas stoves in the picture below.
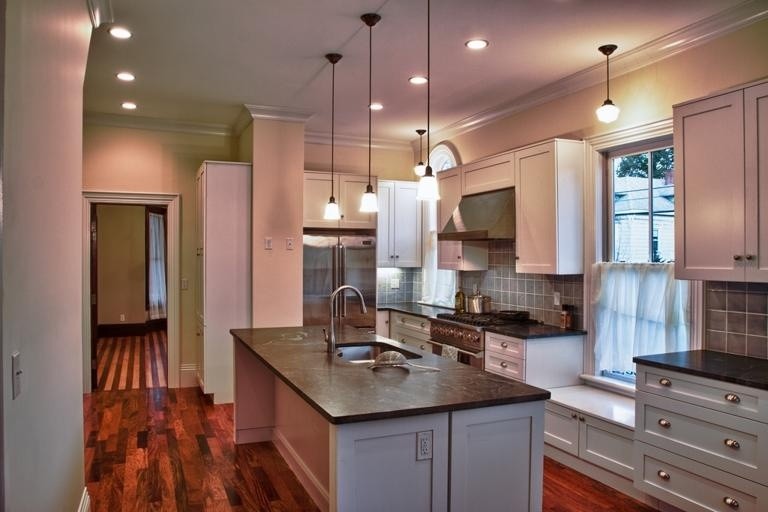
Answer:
[437,311,527,327]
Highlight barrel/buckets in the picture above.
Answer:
[454,287,466,313]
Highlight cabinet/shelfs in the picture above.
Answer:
[672,76,768,283]
[377,180,422,268]
[514,138,584,275]
[632,349,768,512]
[436,165,489,271]
[389,303,456,354]
[544,384,635,499]
[303,170,377,230]
[195,160,251,405]
[483,323,588,390]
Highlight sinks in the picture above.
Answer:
[334,341,423,365]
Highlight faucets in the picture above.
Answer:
[322,286,368,353]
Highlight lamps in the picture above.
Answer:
[416,0,441,202]
[414,129,427,176]
[358,13,382,213]
[324,52,342,220]
[595,44,620,123]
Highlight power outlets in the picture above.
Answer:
[416,430,433,461]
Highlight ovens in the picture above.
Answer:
[425,339,484,370]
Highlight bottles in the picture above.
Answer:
[482,297,491,314]
[455,286,465,315]
[560,304,573,329]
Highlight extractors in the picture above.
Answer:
[438,186,517,242]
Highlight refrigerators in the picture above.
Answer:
[303,235,377,335]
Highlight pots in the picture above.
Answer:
[467,291,490,313]
